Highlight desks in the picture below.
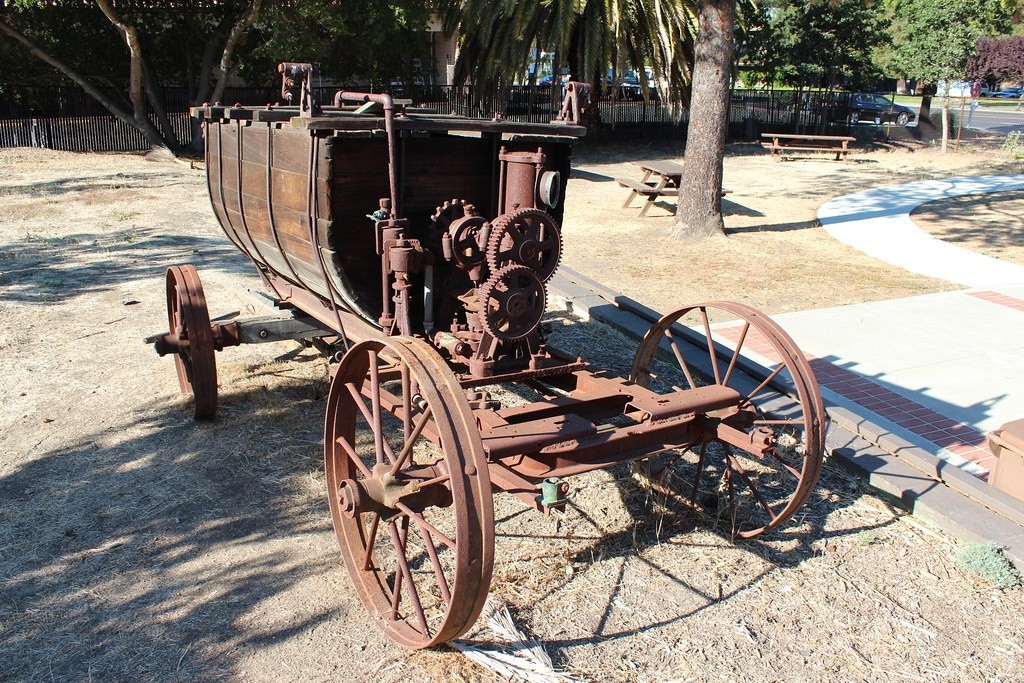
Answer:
[761,133,856,165]
[621,160,684,218]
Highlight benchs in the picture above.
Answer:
[761,142,854,155]
[614,177,733,197]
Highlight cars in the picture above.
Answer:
[643,68,654,80]
[979,81,1024,98]
[599,68,639,85]
[815,92,916,126]
[539,75,563,86]
